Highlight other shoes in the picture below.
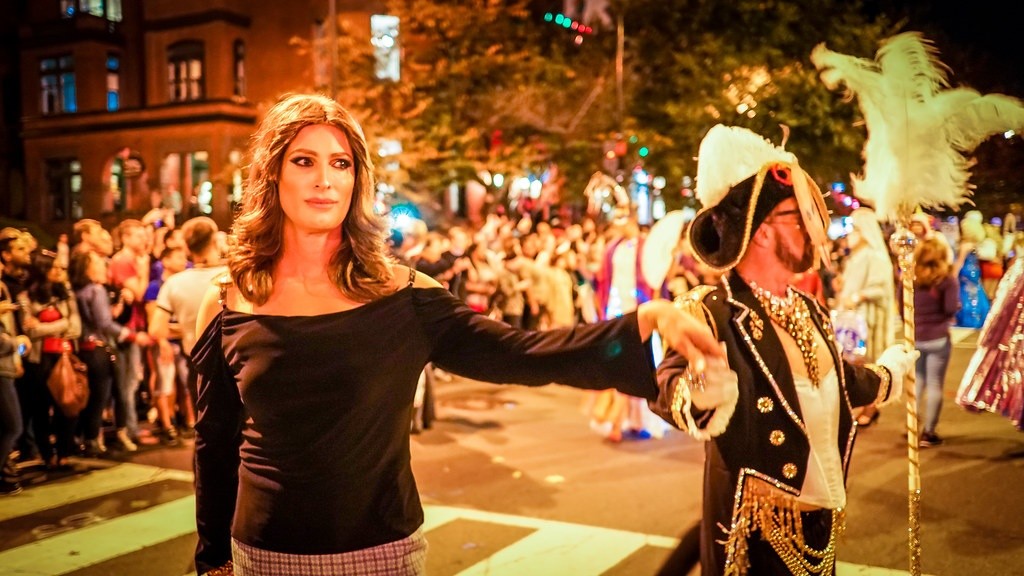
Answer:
[0,427,197,497]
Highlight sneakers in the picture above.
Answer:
[919,433,945,448]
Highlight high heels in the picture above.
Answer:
[857,410,879,428]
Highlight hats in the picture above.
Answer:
[687,124,830,271]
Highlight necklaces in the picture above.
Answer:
[749,277,821,390]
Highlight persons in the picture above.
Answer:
[0,207,235,470]
[789,210,1024,328]
[385,212,727,433]
[149,216,229,489]
[579,204,681,443]
[190,93,730,576]
[647,123,921,576]
[0,261,27,497]
[15,249,82,473]
[898,237,962,448]
[828,207,894,427]
[67,245,152,454]
